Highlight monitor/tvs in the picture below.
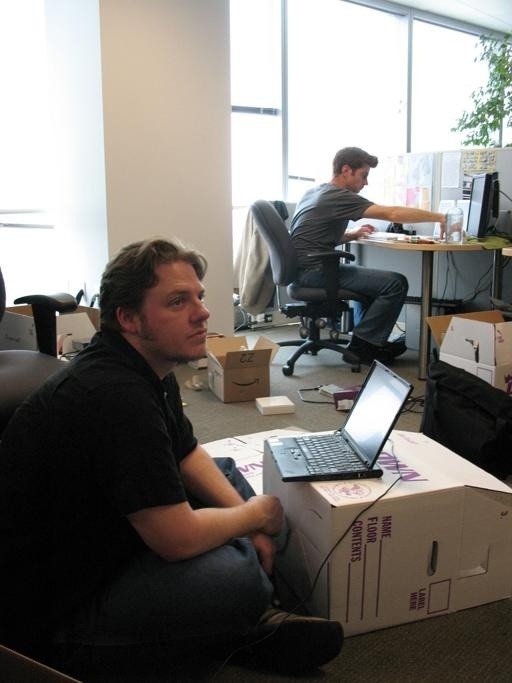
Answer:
[465,174,500,243]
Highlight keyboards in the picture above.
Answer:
[364,231,405,240]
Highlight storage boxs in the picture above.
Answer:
[425,310,512,399]
[201,334,276,404]
[2,306,104,362]
[263,430,512,638]
[199,427,310,497]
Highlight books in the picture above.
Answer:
[361,230,405,241]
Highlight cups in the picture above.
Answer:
[444,212,464,245]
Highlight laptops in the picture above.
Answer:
[268,357,415,482]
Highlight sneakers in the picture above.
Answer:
[228,609,345,672]
[344,344,392,368]
[382,342,406,358]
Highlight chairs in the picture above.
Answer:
[1,267,78,426]
[251,199,364,372]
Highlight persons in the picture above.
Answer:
[286,145,467,366]
[0,232,345,673]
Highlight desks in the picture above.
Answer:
[341,229,512,380]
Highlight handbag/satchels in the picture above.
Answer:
[419,359,512,482]
